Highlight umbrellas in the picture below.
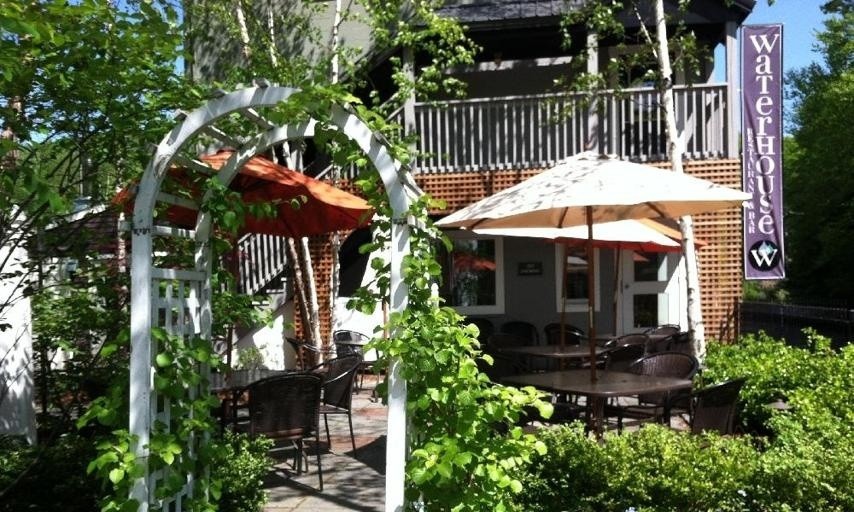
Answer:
[110,148,377,414]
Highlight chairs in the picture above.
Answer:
[221,326,381,494]
[461,317,752,440]
